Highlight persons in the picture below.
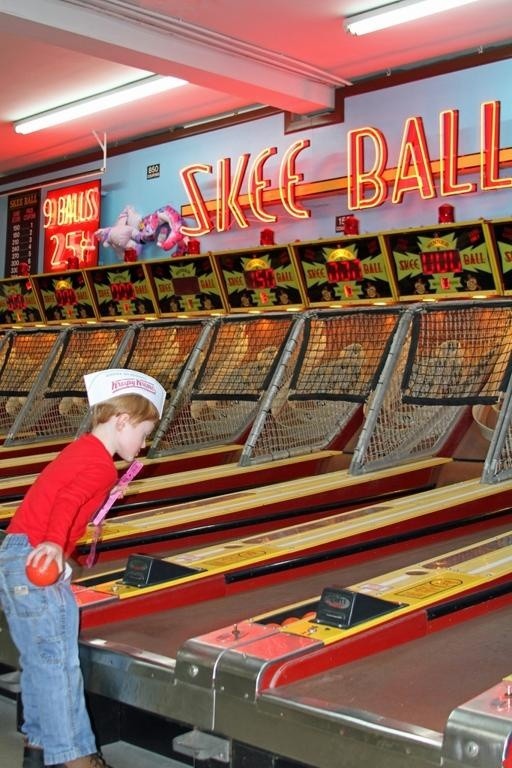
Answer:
[1,368,168,767]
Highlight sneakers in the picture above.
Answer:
[23,745,63,767]
[64,753,112,767]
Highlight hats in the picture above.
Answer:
[82,366,168,421]
